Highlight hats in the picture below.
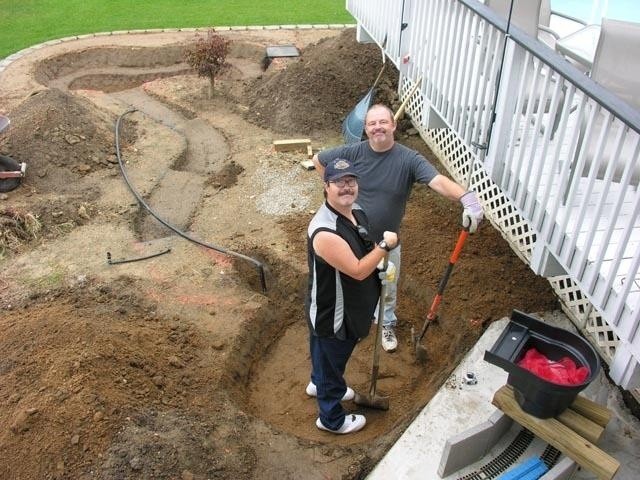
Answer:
[323,157,360,181]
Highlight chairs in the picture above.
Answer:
[461,1,640,212]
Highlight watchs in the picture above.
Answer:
[379,240,390,253]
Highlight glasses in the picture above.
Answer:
[329,178,356,188]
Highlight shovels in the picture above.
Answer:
[355,251,390,411]
[410,216,472,359]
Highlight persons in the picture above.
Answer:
[312,104,485,351]
[305,158,398,435]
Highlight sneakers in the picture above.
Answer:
[315,412,366,434]
[305,379,355,401]
[382,328,398,353]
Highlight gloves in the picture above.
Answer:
[460,190,484,235]
[377,257,397,285]
